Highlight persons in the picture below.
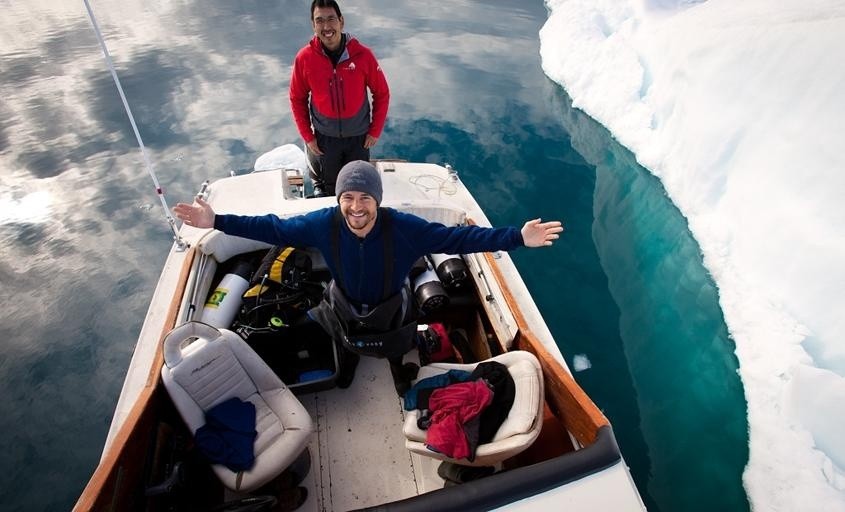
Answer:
[290,0,388,195]
[174,160,565,398]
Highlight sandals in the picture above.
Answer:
[275,486,308,512]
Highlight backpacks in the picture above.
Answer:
[241,243,313,304]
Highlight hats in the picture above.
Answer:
[335,160,383,207]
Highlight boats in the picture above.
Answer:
[71,159,649,512]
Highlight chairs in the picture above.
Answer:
[160,320,315,499]
[402,350,544,485]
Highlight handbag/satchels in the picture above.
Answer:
[207,495,278,512]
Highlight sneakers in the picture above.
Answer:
[391,365,412,400]
[335,353,361,389]
